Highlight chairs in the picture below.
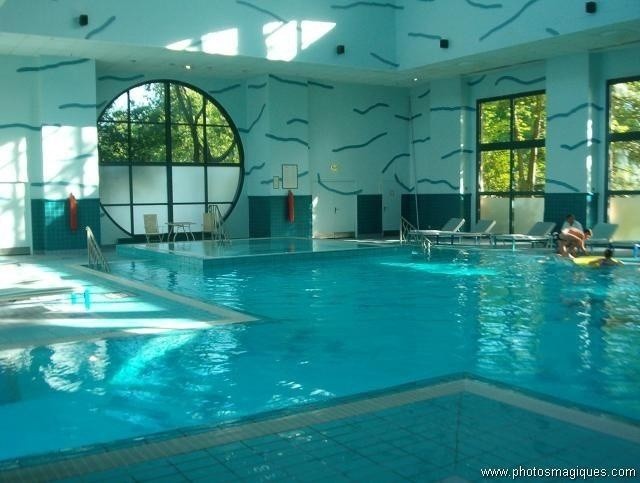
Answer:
[436,218,497,245]
[201,211,218,247]
[489,221,559,251]
[609,238,640,252]
[143,213,163,247]
[576,221,620,256]
[408,216,465,245]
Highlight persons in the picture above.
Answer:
[560,215,585,253]
[589,249,626,266]
[555,226,592,258]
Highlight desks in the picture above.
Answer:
[161,221,197,242]
